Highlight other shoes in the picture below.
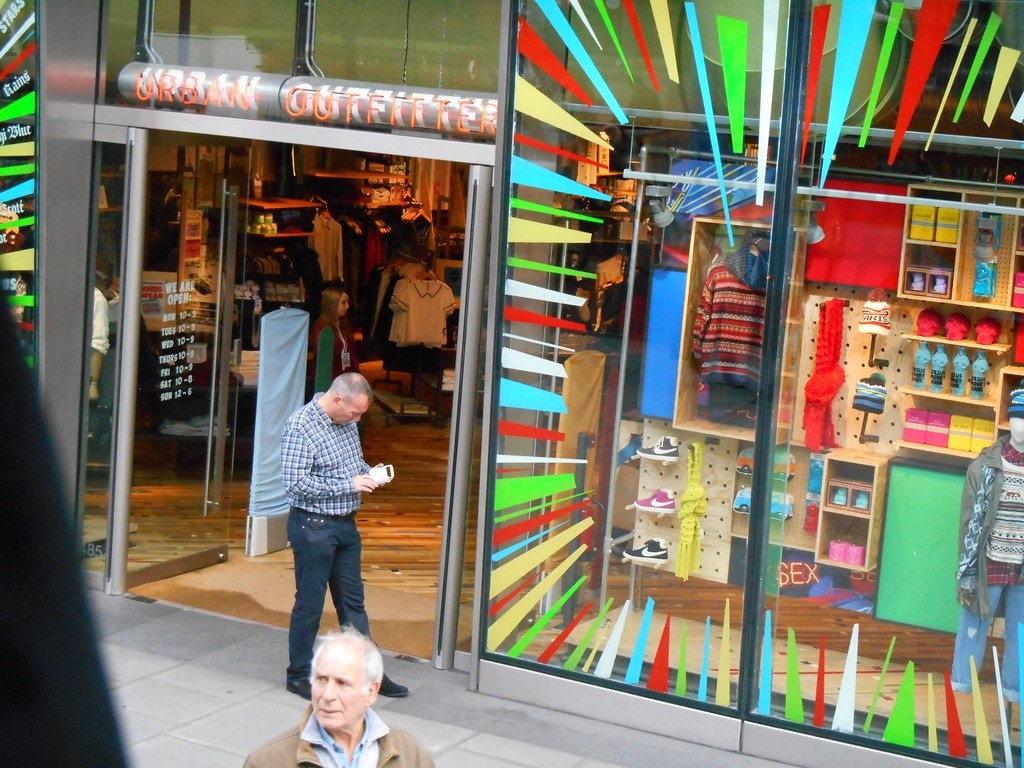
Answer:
[287,678,312,699]
[377,674,409,697]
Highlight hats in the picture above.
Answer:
[852,373,886,414]
[858,288,891,336]
[1007,380,1024,419]
[916,307,1001,345]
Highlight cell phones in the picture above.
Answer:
[368,464,394,484]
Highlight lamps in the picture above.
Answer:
[800,200,825,244]
[645,183,674,228]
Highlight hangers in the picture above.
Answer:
[385,250,438,282]
[304,191,327,205]
[400,201,432,224]
[750,237,762,257]
[335,207,363,234]
[368,209,392,234]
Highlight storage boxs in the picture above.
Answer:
[904,265,935,296]
[970,417,994,453]
[903,407,932,444]
[826,476,860,511]
[926,267,953,298]
[1012,272,1024,308]
[926,410,952,448]
[934,206,959,244]
[947,414,975,451]
[848,479,873,515]
[909,205,938,241]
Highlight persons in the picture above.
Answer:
[89,288,110,398]
[951,381,1024,702]
[243,628,435,768]
[312,288,359,393]
[281,373,409,700]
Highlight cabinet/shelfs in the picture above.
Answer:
[618,183,1024,639]
[10,172,123,213]
[577,210,660,312]
[304,167,410,240]
[238,196,322,237]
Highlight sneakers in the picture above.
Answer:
[633,489,675,514]
[623,539,669,565]
[635,437,680,462]
